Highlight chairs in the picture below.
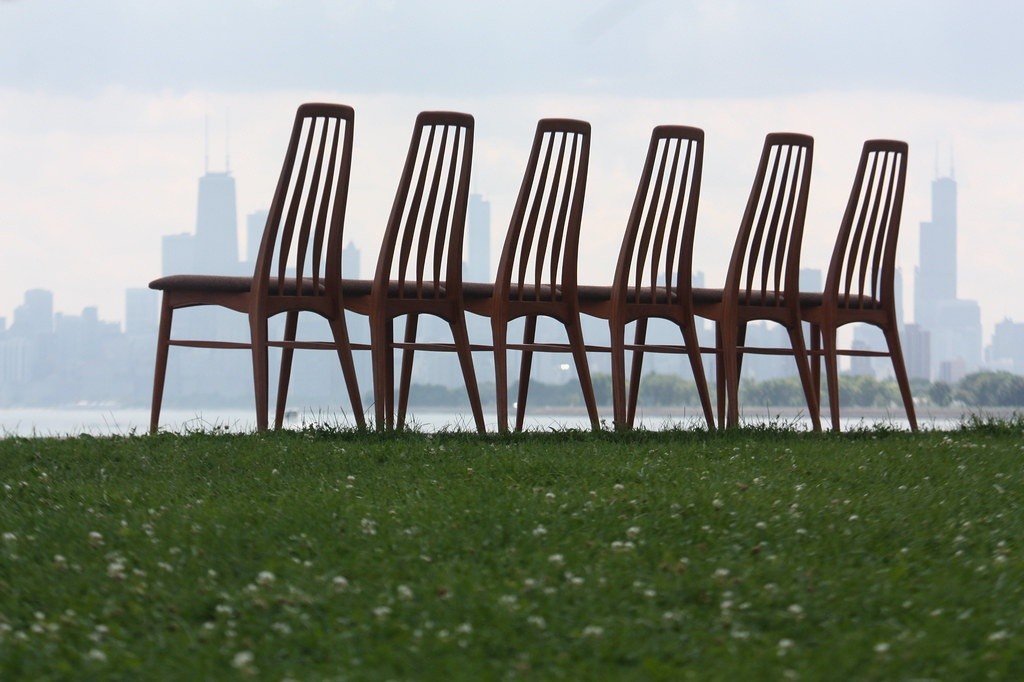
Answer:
[727,140,919,432]
[395,118,601,435]
[278,110,488,433]
[149,102,368,435]
[626,133,822,431]
[515,126,716,431]
